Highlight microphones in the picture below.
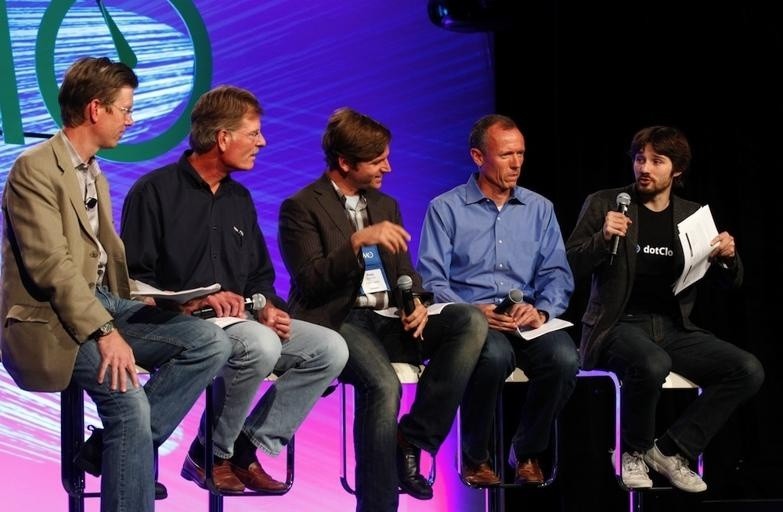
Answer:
[192,292,267,320]
[397,275,423,346]
[493,289,523,314]
[609,192,631,265]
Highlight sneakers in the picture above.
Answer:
[610,446,655,491]
[643,436,707,493]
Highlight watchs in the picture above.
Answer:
[94,321,113,342]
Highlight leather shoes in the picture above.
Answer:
[459,462,502,489]
[229,455,290,494]
[178,451,246,494]
[508,455,545,484]
[76,425,169,501]
[396,433,434,502]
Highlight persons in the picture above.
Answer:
[564,124,765,494]
[121,84,349,494]
[3,55,231,512]
[417,115,581,488]
[275,105,489,511]
